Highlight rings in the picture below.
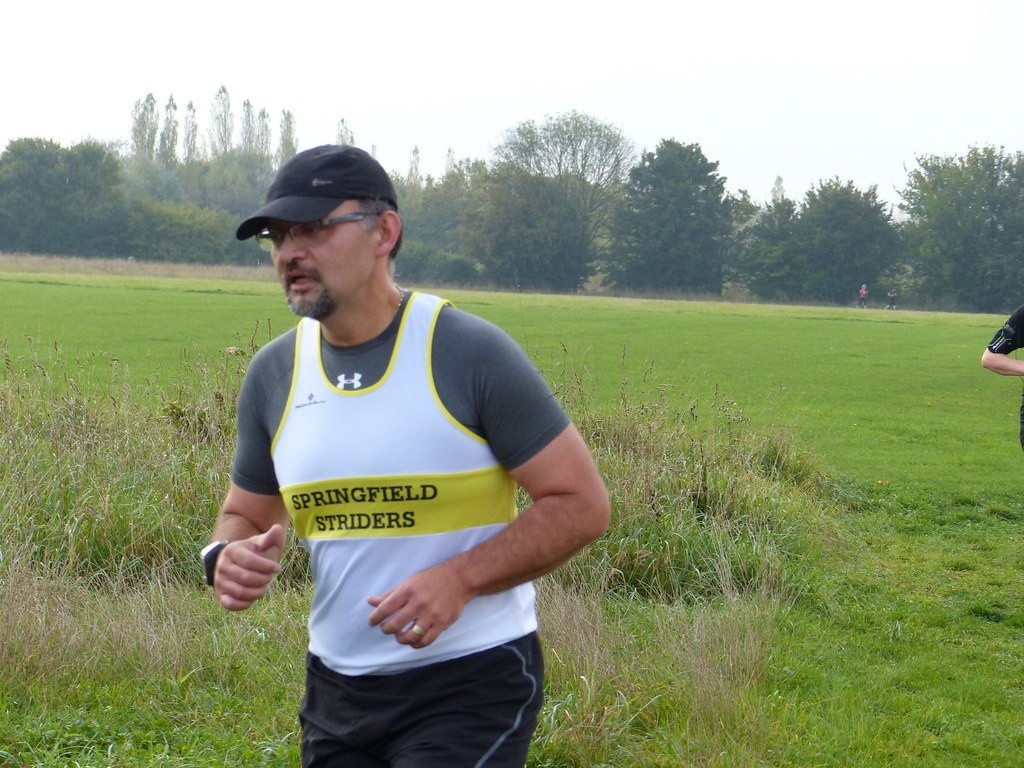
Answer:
[411,623,425,636]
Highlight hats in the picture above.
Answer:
[235,144,398,240]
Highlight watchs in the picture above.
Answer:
[198,539,232,587]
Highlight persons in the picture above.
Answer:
[856,284,868,309]
[208,144,612,768]
[980,303,1024,455]
[884,288,897,311]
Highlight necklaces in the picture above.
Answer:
[395,285,404,316]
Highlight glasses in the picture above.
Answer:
[255,212,379,251]
